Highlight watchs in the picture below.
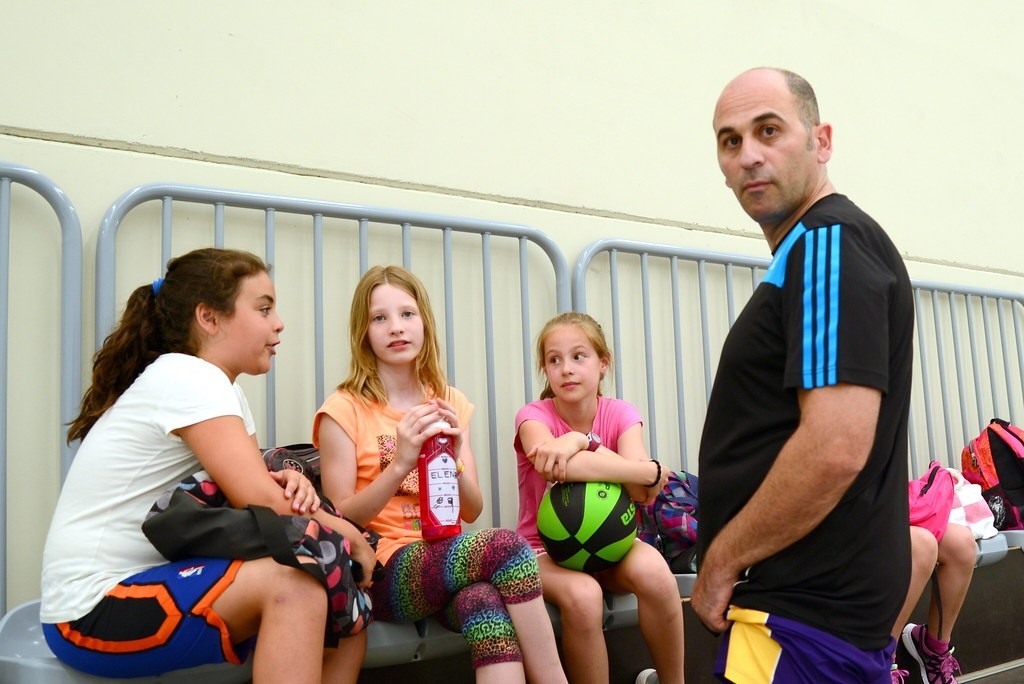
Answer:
[585,431,601,453]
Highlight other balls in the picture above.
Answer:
[536,478,638,571]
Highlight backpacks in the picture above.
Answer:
[142,447,379,639]
[961,419,1024,531]
[908,461,958,543]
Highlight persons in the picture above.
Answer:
[513,311,684,684]
[690,67,915,684]
[38,249,378,684]
[890,522,977,684]
[313,265,568,683]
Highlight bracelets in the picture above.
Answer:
[454,457,465,479]
[643,459,661,488]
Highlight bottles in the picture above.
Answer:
[418,411,462,543]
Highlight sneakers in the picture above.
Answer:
[890,650,910,684]
[902,621,960,684]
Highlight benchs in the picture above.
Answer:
[0,160,1024,684]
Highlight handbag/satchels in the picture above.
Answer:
[634,469,700,572]
